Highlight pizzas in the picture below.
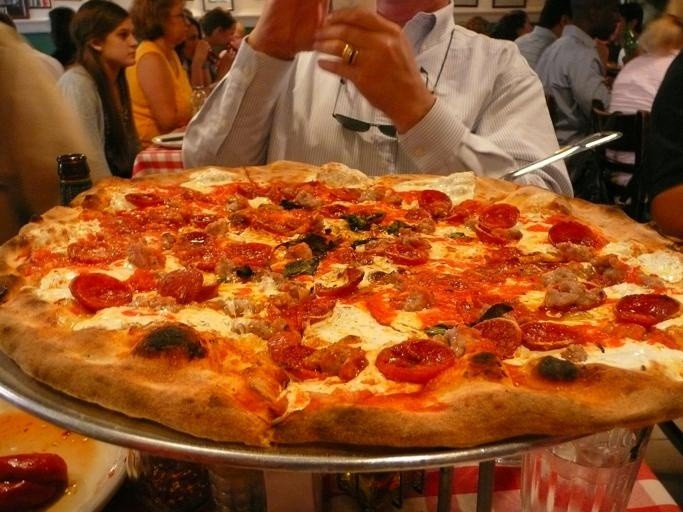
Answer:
[1,160,683,449]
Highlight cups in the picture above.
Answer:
[208,50,218,65]
[519,421,657,512]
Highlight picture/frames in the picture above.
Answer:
[1,0,32,22]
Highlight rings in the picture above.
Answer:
[343,43,359,64]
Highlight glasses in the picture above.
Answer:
[331,111,397,137]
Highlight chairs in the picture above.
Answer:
[592,97,651,223]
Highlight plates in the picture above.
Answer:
[150,133,186,150]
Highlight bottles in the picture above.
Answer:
[192,86,206,119]
[56,152,94,208]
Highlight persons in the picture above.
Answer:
[639,50,683,238]
[0,20,104,247]
[179,0,576,199]
[515,1,682,222]
[175,13,212,89]
[200,6,237,96]
[48,8,79,66]
[603,17,682,224]
[467,10,532,42]
[51,1,142,185]
[219,22,246,58]
